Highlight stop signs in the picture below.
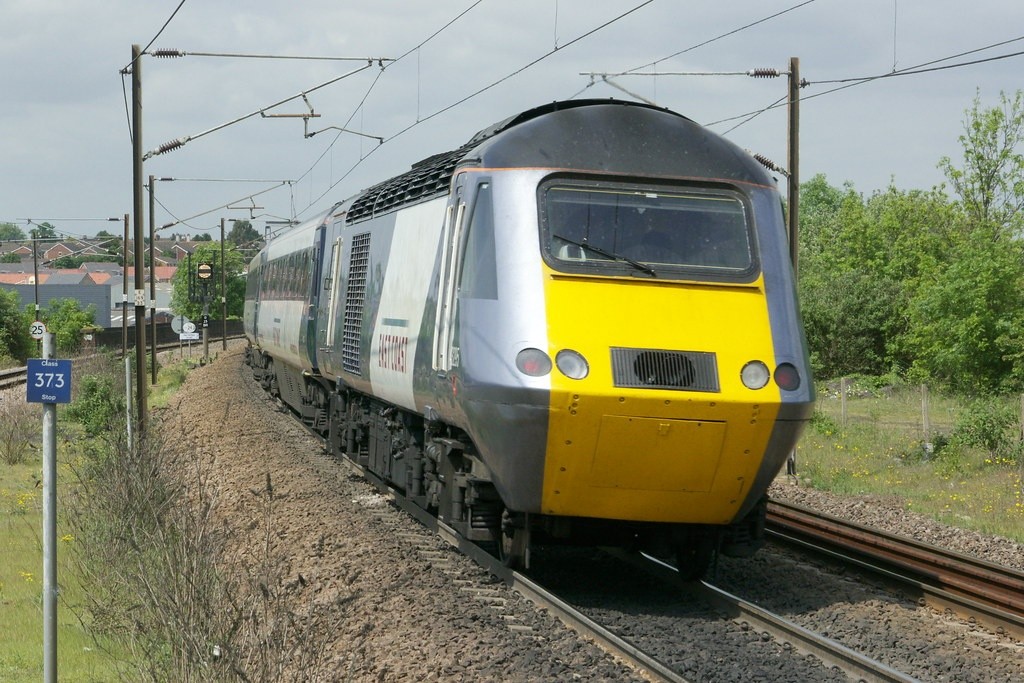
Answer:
[198,264,213,279]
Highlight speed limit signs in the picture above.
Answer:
[28,321,46,340]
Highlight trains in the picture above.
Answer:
[243,99,815,578]
